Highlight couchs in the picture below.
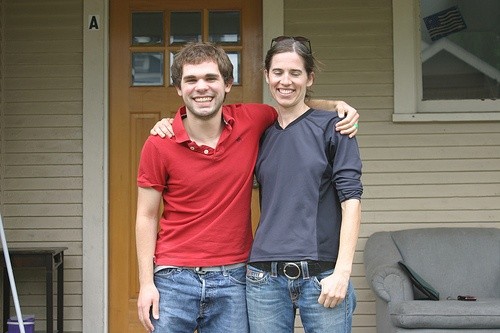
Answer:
[363,227,500,333]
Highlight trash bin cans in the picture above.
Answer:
[7,316,35,333]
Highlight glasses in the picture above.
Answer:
[270,36,311,54]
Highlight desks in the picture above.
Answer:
[0,246,69,333]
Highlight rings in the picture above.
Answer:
[354,124,359,128]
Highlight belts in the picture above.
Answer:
[154,261,246,274]
[249,257,336,280]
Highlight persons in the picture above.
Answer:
[135,41,360,333]
[150,36,364,333]
